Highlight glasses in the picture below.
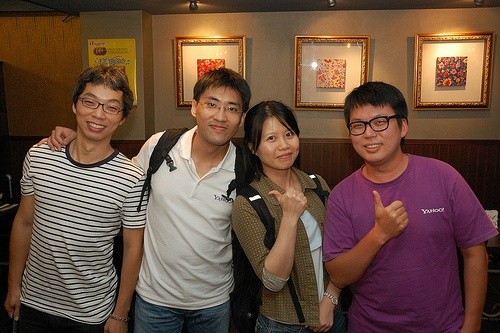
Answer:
[197,100,244,118]
[347,114,403,136]
[78,96,124,115]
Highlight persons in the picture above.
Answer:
[322,81,497,333]
[38,67,252,333]
[4,66,150,333]
[232,101,342,333]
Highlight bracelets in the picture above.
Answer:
[109,314,130,321]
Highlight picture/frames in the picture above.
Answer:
[173,35,246,110]
[293,35,371,110]
[412,32,495,111]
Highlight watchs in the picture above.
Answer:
[323,292,341,306]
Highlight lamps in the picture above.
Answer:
[189,0,198,10]
[473,0,484,6]
[327,0,336,7]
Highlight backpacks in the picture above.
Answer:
[232,172,329,333]
